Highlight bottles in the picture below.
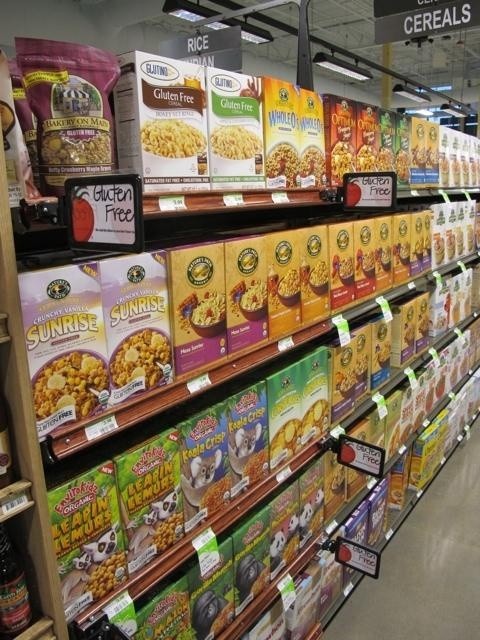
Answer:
[0,524,32,634]
[299,258,309,280]
[267,263,278,288]
[0,390,12,489]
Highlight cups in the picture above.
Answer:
[184,75,201,88]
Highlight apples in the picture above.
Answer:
[337,540,352,563]
[347,176,362,206]
[340,439,355,464]
[71,186,94,241]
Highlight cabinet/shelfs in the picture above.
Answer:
[0,132,480,640]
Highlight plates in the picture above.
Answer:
[111,328,172,391]
[30,350,110,415]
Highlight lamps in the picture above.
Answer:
[162,0,273,45]
[393,79,431,104]
[440,100,469,118]
[313,48,373,81]
[417,109,434,117]
[397,106,440,116]
[413,85,452,93]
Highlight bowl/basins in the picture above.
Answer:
[361,257,375,276]
[414,251,423,258]
[238,291,267,320]
[188,300,224,338]
[275,278,301,306]
[310,268,331,295]
[400,254,410,263]
[380,259,391,270]
[338,262,353,284]
